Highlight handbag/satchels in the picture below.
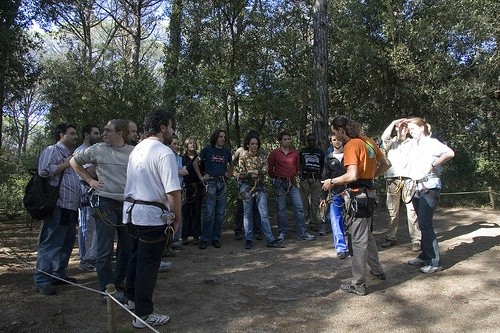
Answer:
[344,188,375,218]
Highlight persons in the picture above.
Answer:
[321,115,391,296]
[34,120,138,294]
[137,126,203,269]
[123,108,183,326]
[379,118,422,251]
[395,117,454,273]
[267,132,316,242]
[300,133,353,258]
[193,129,233,250]
[227,130,285,249]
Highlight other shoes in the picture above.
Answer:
[200,241,207,249]
[236,233,243,241]
[408,257,431,266]
[182,237,190,245]
[381,238,397,247]
[267,240,285,248]
[337,252,347,259]
[341,282,366,295]
[412,243,421,252]
[193,236,200,245]
[245,240,252,249]
[34,284,57,295]
[172,245,185,250]
[158,262,172,271]
[278,232,288,240]
[297,231,317,241]
[212,239,219,248]
[51,276,77,285]
[419,265,443,274]
[364,269,385,280]
[78,262,98,271]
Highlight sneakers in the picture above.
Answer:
[133,311,170,328]
[123,298,137,309]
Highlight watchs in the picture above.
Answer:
[331,178,333,184]
[431,161,435,167]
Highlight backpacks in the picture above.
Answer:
[23,146,68,221]
